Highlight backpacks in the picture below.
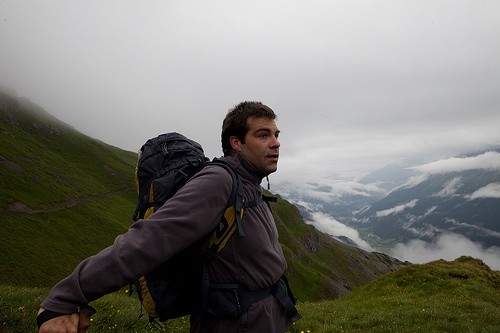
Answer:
[128,132,277,330]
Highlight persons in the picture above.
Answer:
[36,102,301,333]
